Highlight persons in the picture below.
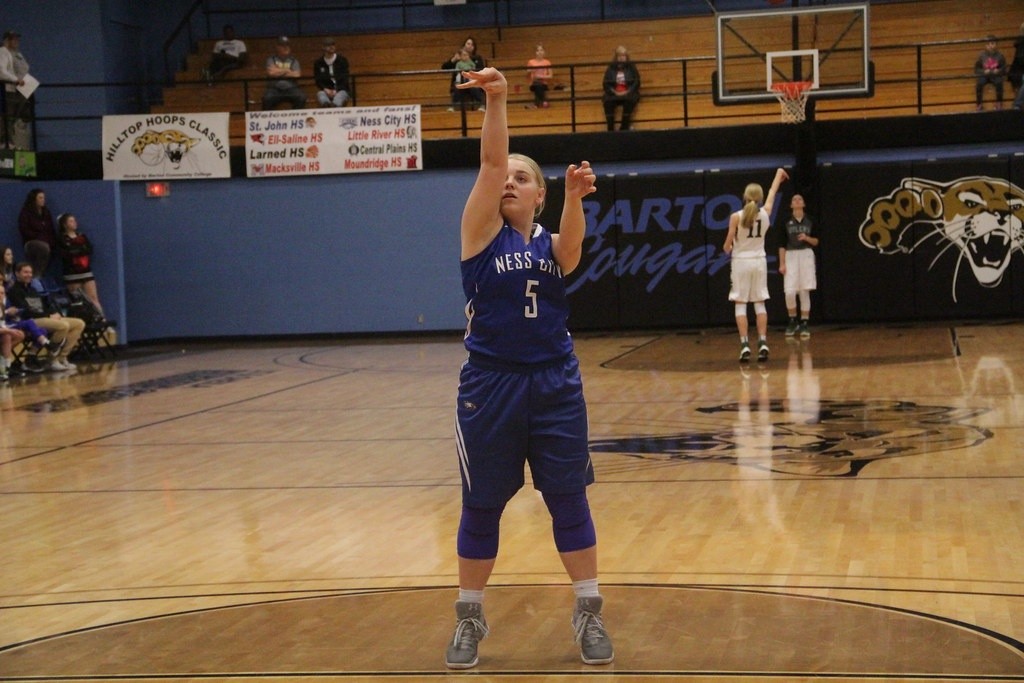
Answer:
[200,22,250,88]
[600,46,641,133]
[973,34,1007,110]
[0,186,107,382]
[442,67,613,669]
[1006,20,1024,110]
[0,29,37,168]
[722,167,790,364]
[442,36,485,112]
[522,43,553,110]
[778,193,819,336]
[261,35,306,110]
[313,37,357,107]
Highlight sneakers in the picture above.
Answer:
[785,337,799,346]
[445,600,490,669]
[785,319,800,335]
[757,342,769,359]
[799,322,810,336]
[739,343,751,361]
[571,594,614,664]
[756,363,770,379]
[801,337,810,344]
[740,364,751,378]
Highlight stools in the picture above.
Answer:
[149,0,1024,148]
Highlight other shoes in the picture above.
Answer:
[203,69,213,86]
[19,370,77,384]
[0,360,77,378]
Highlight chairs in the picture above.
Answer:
[0,284,117,379]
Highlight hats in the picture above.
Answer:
[4,29,21,38]
[277,35,290,44]
[322,36,335,45]
